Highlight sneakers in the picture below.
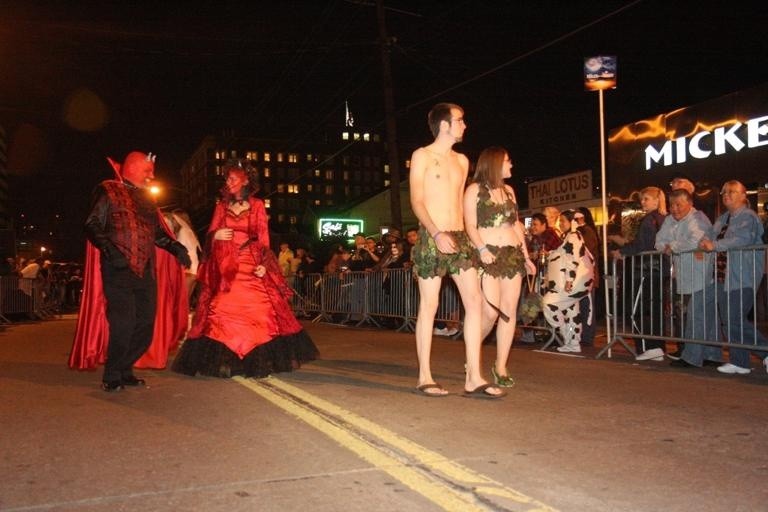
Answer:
[557,343,583,354]
[719,363,756,377]
[670,356,704,369]
[492,363,515,389]
[636,348,662,363]
[699,357,726,376]
[652,355,662,363]
[433,327,458,336]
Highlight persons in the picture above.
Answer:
[666,176,695,197]
[517,193,600,353]
[0,255,81,324]
[408,104,509,399]
[462,144,538,388]
[204,162,288,378]
[654,189,725,369]
[78,151,191,392]
[605,186,669,362]
[274,229,418,328]
[698,178,767,375]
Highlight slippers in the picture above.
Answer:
[412,383,450,399]
[464,382,507,399]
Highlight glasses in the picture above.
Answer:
[718,188,741,195]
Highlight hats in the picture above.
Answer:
[383,229,400,240]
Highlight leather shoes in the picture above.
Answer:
[126,374,145,387]
[101,381,126,394]
